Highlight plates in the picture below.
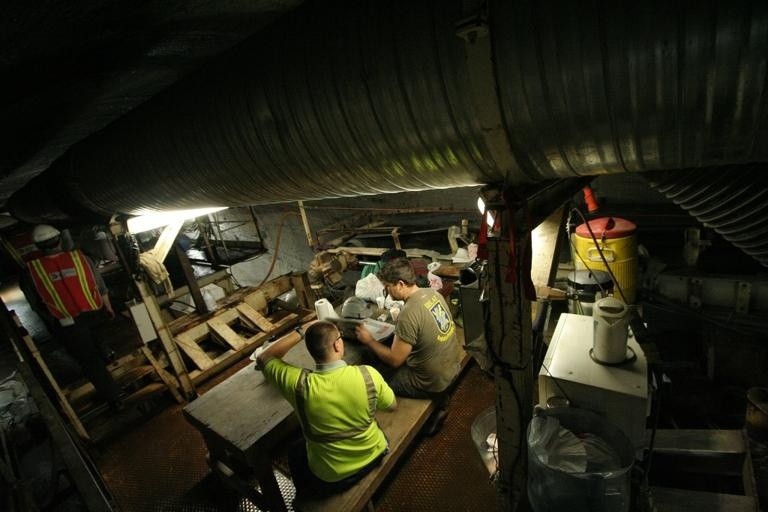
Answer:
[588,344,637,367]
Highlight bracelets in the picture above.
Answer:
[294,326,305,339]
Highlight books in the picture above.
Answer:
[325,318,396,343]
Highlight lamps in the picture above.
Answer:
[475,195,496,228]
[128,207,231,236]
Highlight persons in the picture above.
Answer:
[354,257,467,435]
[256,322,398,495]
[19,224,125,414]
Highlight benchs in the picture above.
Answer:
[297,392,434,512]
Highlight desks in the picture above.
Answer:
[183,299,394,512]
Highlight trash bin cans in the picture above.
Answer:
[527,407,635,512]
[469,406,499,483]
[746,386,768,477]
[568,269,614,316]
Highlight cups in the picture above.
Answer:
[389,308,400,322]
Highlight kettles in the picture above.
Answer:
[593,294,630,363]
